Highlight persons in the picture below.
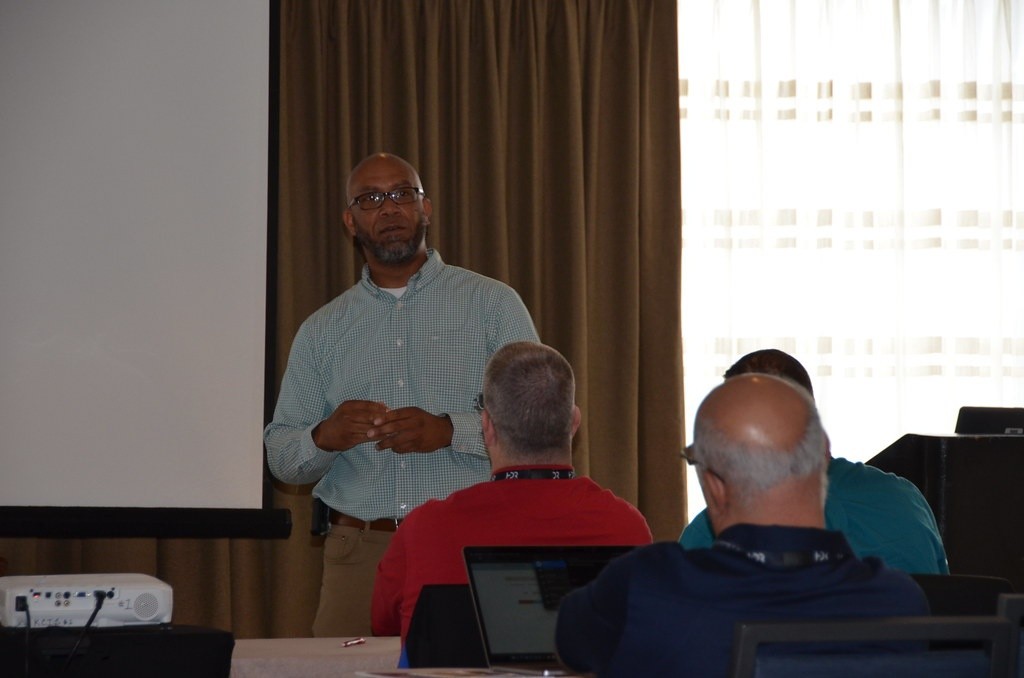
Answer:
[371,342,655,670]
[265,152,543,637]
[555,375,930,678]
[675,349,953,581]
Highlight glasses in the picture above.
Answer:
[349,187,425,211]
[680,444,724,484]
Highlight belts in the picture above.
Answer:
[332,510,403,533]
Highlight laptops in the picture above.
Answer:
[464,546,638,678]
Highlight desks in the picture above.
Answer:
[225,632,408,675]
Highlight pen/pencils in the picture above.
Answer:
[342,638,366,647]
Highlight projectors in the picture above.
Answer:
[0,573,174,629]
